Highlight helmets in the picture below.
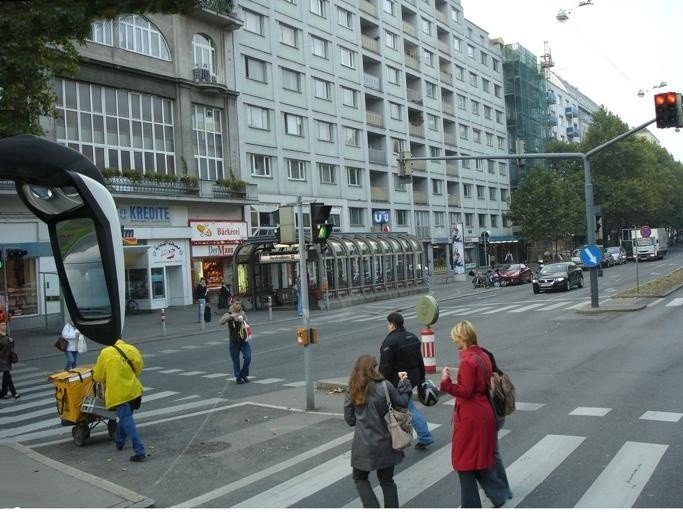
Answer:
[416,379,440,407]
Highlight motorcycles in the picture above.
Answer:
[468,269,506,288]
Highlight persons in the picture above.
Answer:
[344,353,412,508]
[132,283,140,300]
[295,274,302,317]
[379,312,435,450]
[92,339,147,462]
[0,302,5,324]
[140,280,147,300]
[454,248,460,263]
[16,287,28,310]
[505,249,514,264]
[441,320,508,508]
[194,277,209,322]
[0,320,21,399]
[477,346,513,499]
[61,317,82,372]
[218,296,252,385]
[214,284,232,316]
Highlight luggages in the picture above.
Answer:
[204,306,211,322]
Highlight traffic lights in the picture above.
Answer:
[5,248,28,259]
[654,92,682,129]
[309,202,333,243]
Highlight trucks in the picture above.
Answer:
[630,227,670,262]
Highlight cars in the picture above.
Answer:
[496,263,533,285]
[531,262,583,293]
[537,244,627,271]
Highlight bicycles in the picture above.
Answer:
[124,294,138,315]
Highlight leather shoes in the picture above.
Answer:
[3,395,10,399]
[14,395,21,398]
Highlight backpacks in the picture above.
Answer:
[130,395,142,410]
[474,347,516,418]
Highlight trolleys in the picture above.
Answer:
[47,365,127,449]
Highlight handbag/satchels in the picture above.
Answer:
[382,379,416,452]
[239,320,252,342]
[54,337,69,352]
[11,352,18,364]
[77,333,88,354]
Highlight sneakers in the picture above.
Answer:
[240,369,250,382]
[129,453,147,463]
[414,440,435,450]
[236,378,244,384]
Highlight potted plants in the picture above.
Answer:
[227,166,246,199]
